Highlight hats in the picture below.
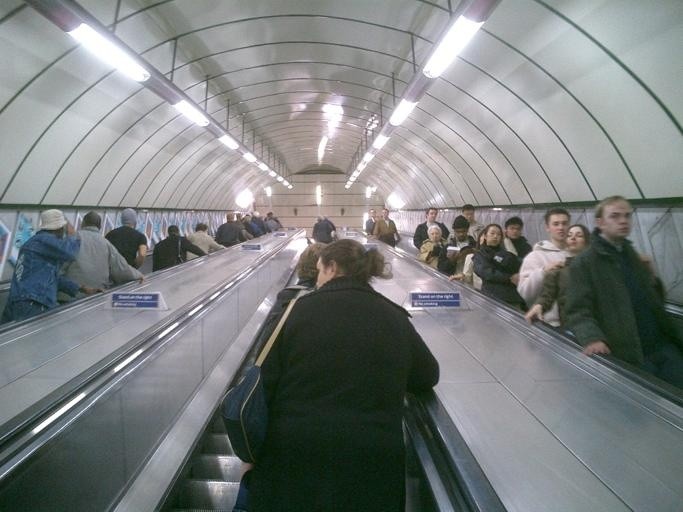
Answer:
[38,209,69,232]
[227,214,234,222]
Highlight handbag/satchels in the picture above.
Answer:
[218,364,273,466]
[175,238,184,265]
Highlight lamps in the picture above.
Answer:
[30,0,295,191]
[343,0,507,191]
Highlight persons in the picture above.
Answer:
[517,209,571,328]
[232,243,439,510]
[527,224,653,334]
[58,212,145,304]
[313,216,335,242]
[105,207,146,271]
[216,211,282,248]
[413,204,533,313]
[153,226,207,272]
[5,208,106,322]
[575,194,681,386]
[366,208,401,247]
[186,223,226,260]
